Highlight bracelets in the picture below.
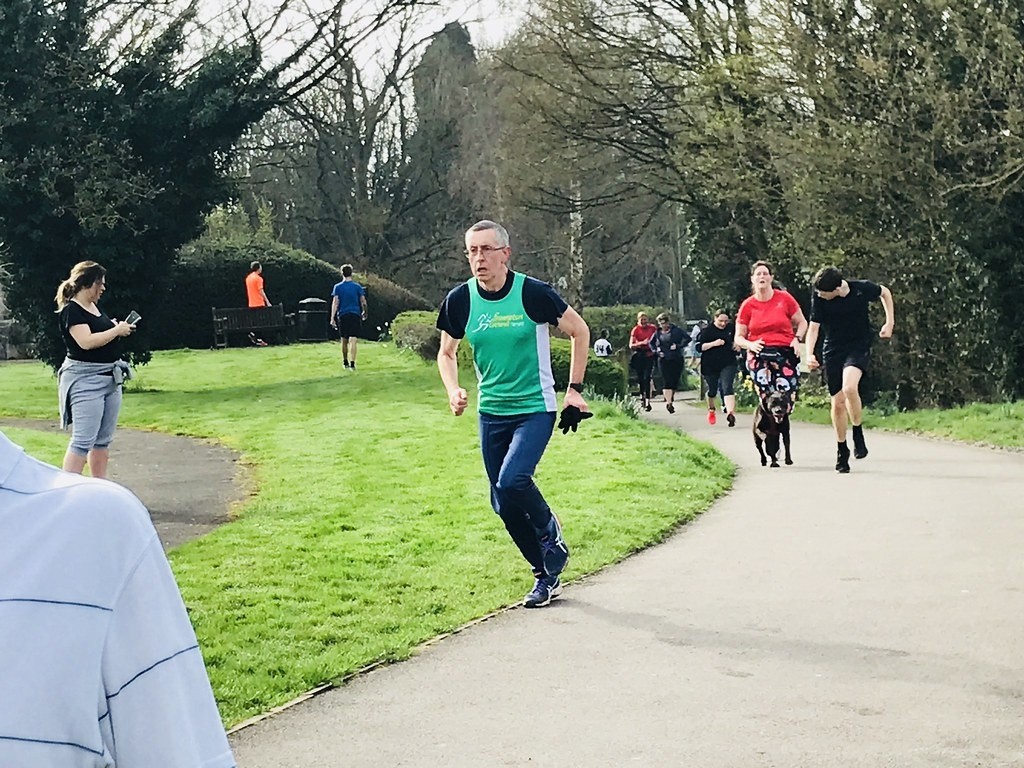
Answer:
[569,383,585,393]
[795,335,802,343]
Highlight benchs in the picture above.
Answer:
[211,303,296,348]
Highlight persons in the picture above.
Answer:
[435,220,590,608]
[649,313,692,414]
[330,264,368,370]
[629,311,657,412]
[734,260,808,460]
[0,431,238,768]
[594,330,612,358]
[245,261,272,345]
[53,260,137,479]
[690,311,713,340]
[805,266,894,474]
[695,307,741,427]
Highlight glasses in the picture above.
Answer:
[463,246,505,258]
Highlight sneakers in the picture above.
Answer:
[852,429,868,459]
[350,363,356,370]
[708,410,716,424]
[727,414,735,427]
[540,512,569,575]
[836,446,851,472]
[343,361,350,369]
[249,332,256,344]
[721,404,727,414]
[524,575,563,608]
[666,403,675,413]
[256,339,268,346]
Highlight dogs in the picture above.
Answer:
[752,393,793,467]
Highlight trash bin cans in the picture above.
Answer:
[299,298,328,343]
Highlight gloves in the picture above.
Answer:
[558,405,593,435]
